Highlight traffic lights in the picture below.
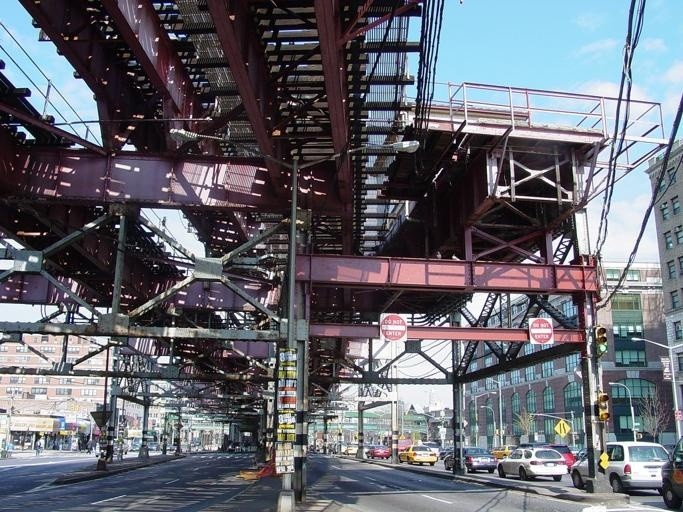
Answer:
[599,392,610,421]
[596,327,608,355]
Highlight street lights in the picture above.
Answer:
[608,381,636,442]
[163,126,421,511]
[439,409,446,449]
[630,337,683,439]
[479,405,496,446]
[473,391,496,448]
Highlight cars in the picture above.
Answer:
[567,445,588,460]
[542,444,576,474]
[659,435,683,508]
[496,448,568,481]
[94,438,200,459]
[516,443,545,451]
[569,441,672,492]
[226,442,240,452]
[442,447,497,474]
[489,444,516,460]
[308,436,454,467]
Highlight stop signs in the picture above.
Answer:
[380,313,408,341]
[529,318,554,345]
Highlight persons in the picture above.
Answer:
[34,438,123,460]
